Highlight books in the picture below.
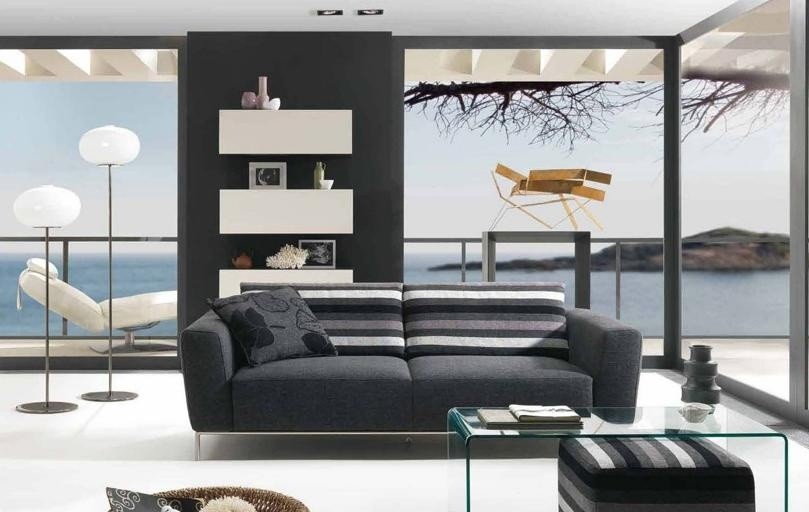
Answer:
[509,403,582,421]
[476,408,583,427]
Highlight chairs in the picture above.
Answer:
[18,257,178,354]
[106,486,311,512]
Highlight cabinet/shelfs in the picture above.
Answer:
[219,110,353,300]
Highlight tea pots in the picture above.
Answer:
[230,253,256,270]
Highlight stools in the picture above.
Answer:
[558,429,756,512]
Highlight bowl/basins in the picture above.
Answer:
[318,179,334,190]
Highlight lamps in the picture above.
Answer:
[13,186,81,414]
[78,124,141,402]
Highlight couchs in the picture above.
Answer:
[178,280,643,461]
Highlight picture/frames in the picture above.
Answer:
[298,239,336,269]
[249,162,287,189]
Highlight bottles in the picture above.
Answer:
[240,91,258,110]
[313,161,327,189]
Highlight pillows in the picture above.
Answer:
[27,257,58,280]
[106,487,203,512]
[203,285,339,368]
